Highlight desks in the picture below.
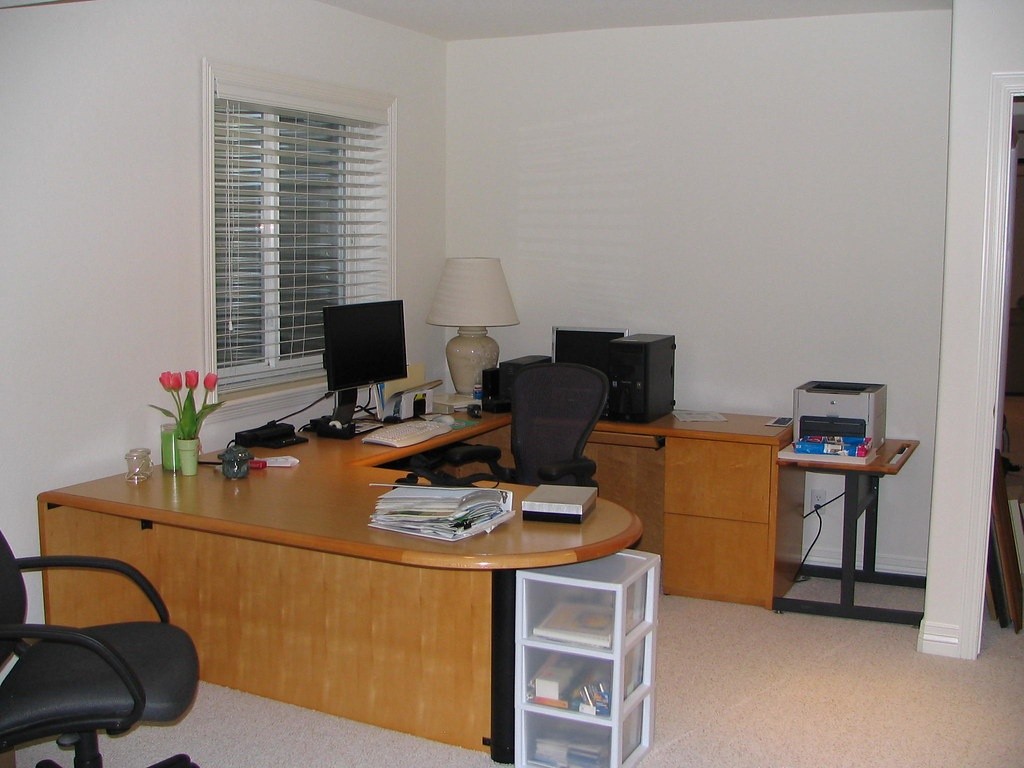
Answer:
[771,438,924,626]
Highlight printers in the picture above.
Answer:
[793,380,886,452]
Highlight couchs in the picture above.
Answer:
[1004,308,1024,396]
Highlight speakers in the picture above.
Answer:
[500,355,552,400]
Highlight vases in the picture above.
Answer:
[176,438,200,476]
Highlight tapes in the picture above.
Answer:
[329,420,342,429]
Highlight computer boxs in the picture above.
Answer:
[609,334,676,422]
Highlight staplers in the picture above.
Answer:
[467,403,482,419]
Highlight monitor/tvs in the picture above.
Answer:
[551,325,629,378]
[310,300,408,436]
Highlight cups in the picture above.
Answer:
[160,424,181,473]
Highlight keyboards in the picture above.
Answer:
[362,421,452,447]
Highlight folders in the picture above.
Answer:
[367,482,515,542]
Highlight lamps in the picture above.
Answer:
[424,256,520,396]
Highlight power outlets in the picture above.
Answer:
[810,487,826,515]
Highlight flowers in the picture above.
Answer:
[148,368,228,440]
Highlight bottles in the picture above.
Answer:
[474,371,483,400]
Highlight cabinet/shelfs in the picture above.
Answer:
[515,548,660,768]
[37,410,807,764]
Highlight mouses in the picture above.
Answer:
[432,415,455,426]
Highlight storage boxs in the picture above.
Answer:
[536,662,583,700]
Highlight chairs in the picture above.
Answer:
[0,531,200,768]
[447,362,611,496]
